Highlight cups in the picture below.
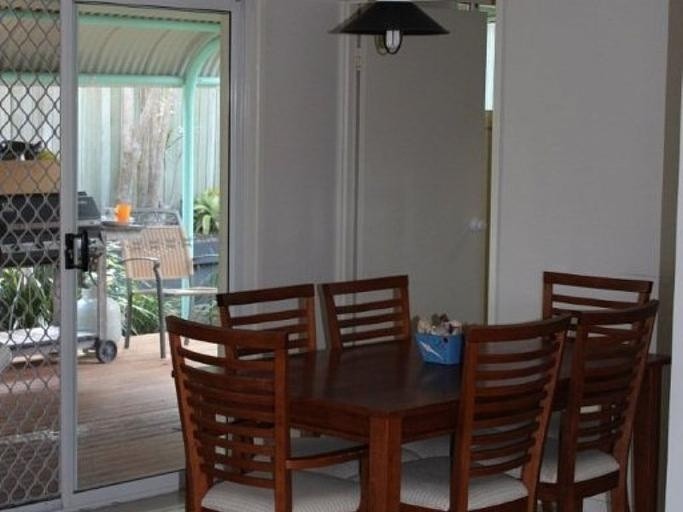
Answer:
[114,203,130,224]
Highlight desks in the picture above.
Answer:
[169,336,672,510]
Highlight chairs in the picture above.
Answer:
[105,205,217,358]
[164,313,367,510]
[538,269,656,350]
[506,298,658,511]
[315,272,414,349]
[391,312,576,512]
[214,283,320,473]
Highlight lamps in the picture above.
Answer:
[333,0,450,54]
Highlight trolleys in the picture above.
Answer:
[0,196,144,374]
